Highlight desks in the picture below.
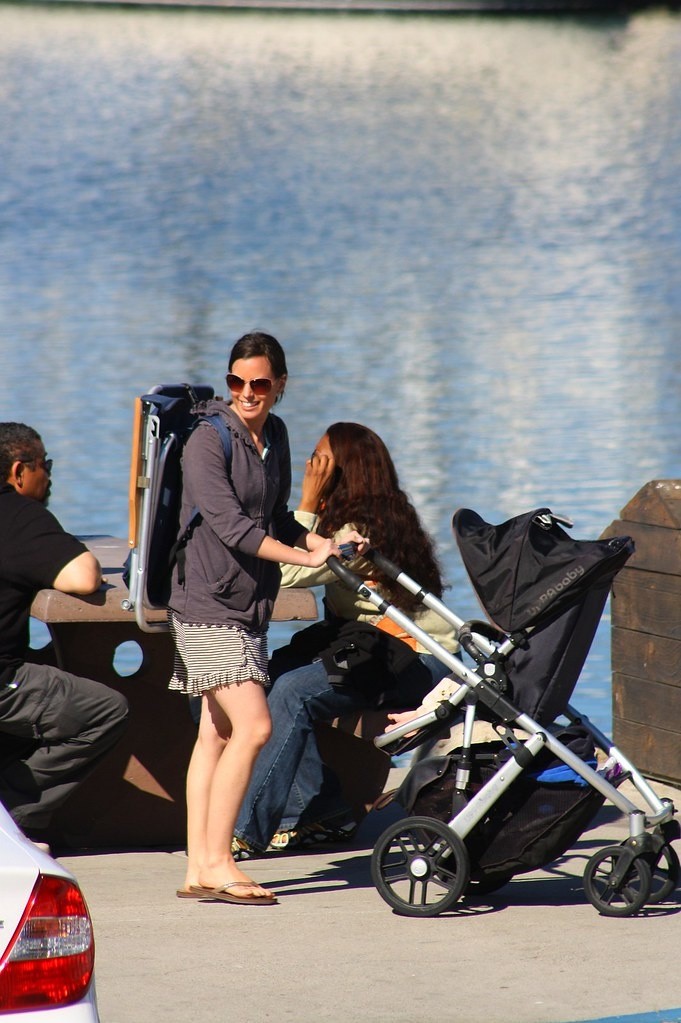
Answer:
[22,529,320,850]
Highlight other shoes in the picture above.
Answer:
[230,836,262,862]
[269,820,359,849]
[27,819,95,850]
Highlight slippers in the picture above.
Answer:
[177,889,212,898]
[189,879,278,905]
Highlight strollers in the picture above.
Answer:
[326,506,681,919]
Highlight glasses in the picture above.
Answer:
[226,373,280,396]
[25,459,53,477]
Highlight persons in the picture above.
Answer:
[167,331,370,904]
[0,423,130,854]
[229,422,462,861]
